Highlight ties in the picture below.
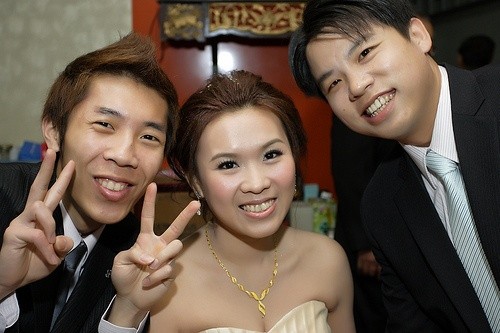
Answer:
[426,150,500,333]
[49,242,87,333]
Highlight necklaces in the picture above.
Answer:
[204,220,279,319]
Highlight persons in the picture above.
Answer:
[148,69,356,333]
[288,0,500,333]
[0,30,202,333]
